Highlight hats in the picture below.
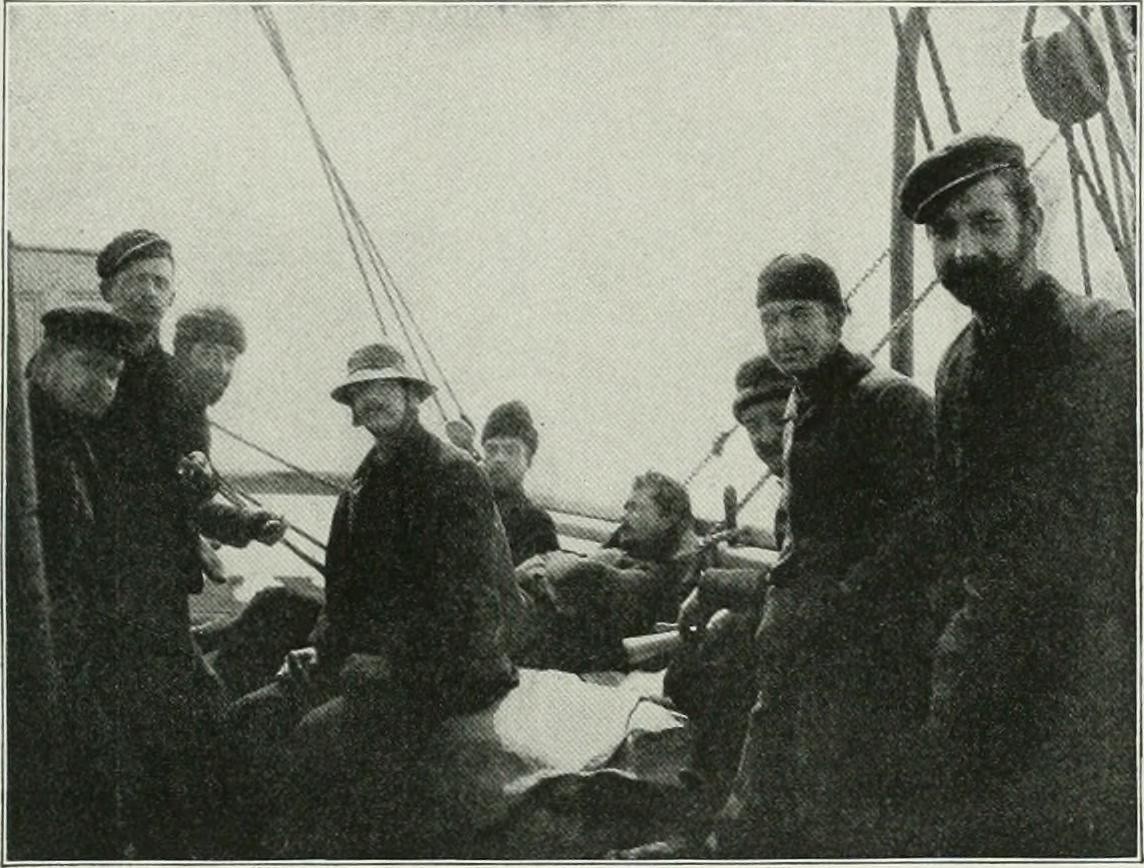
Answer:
[330,344,438,405]
[899,129,1023,224]
[756,252,843,305]
[733,354,794,422]
[40,302,144,358]
[96,229,165,278]
[479,400,539,454]
[175,306,247,356]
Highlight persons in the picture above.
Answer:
[190,400,691,703]
[899,134,1135,858]
[22,229,291,859]
[655,253,934,858]
[222,342,524,859]
